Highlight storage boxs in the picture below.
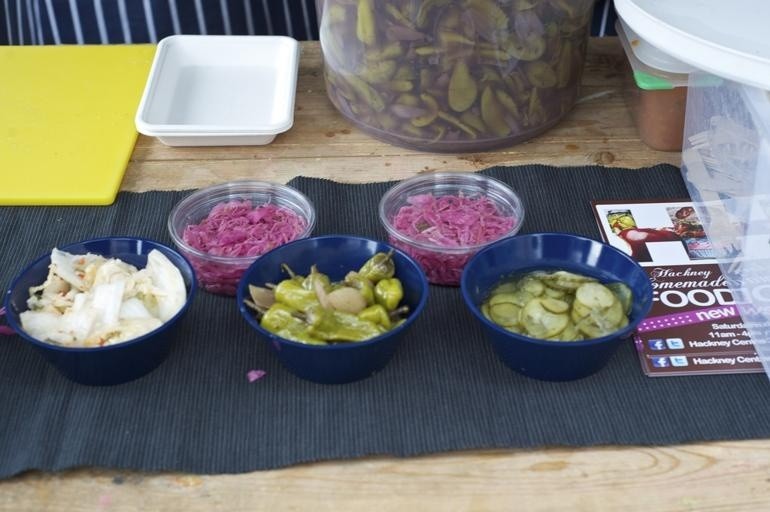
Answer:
[678,62,769,388]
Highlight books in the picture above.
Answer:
[590,197,770,377]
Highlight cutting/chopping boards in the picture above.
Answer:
[0,37,160,209]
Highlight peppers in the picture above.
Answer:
[259,252,403,346]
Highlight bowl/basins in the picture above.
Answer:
[463,230,652,379]
[234,232,428,383]
[135,33,298,148]
[374,174,523,284]
[4,236,194,384]
[168,180,317,296]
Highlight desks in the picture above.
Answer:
[0,36,769,512]
